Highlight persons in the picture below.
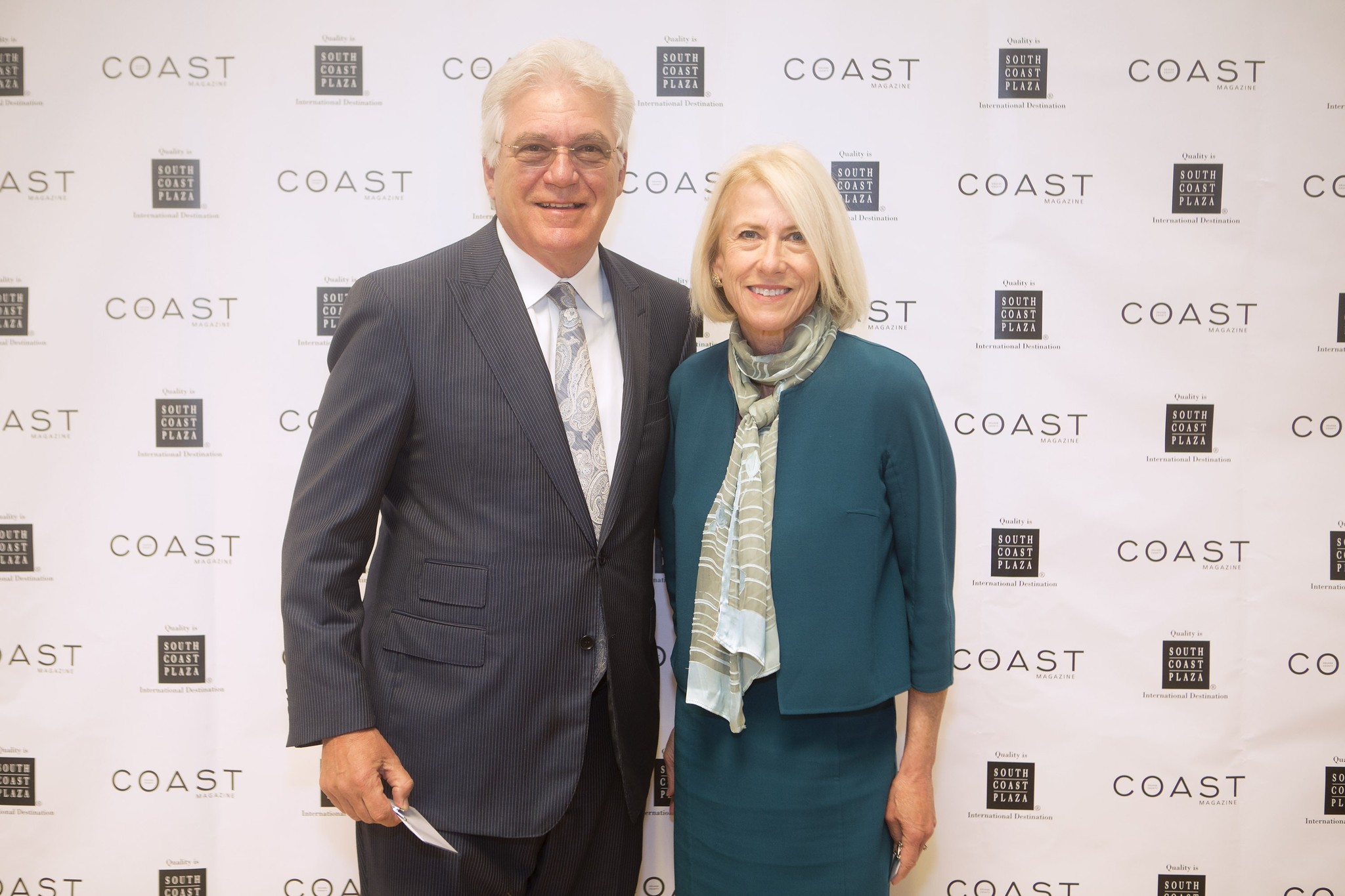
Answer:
[654,150,957,896]
[280,44,705,896]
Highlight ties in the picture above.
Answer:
[548,282,611,695]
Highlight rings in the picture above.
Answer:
[923,845,928,851]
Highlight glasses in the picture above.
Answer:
[490,139,622,171]
